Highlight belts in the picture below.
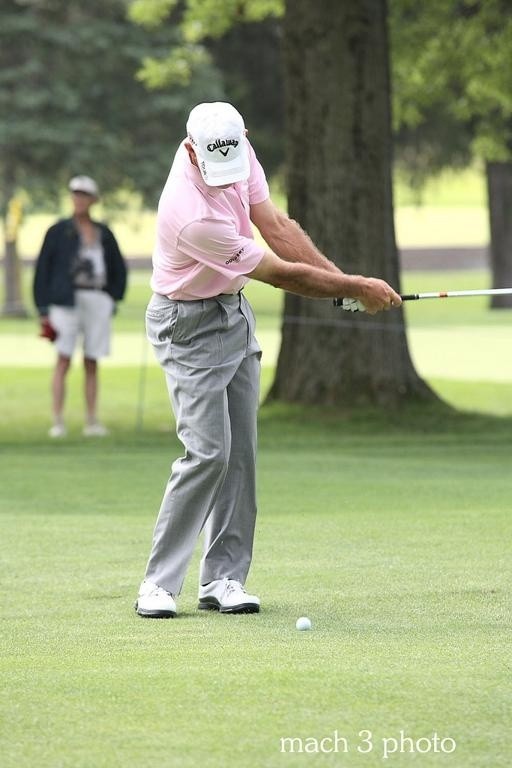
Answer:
[74,282,107,292]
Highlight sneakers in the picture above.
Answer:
[49,423,107,438]
[197,577,260,613]
[135,580,178,619]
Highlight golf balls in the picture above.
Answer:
[296,618,311,633]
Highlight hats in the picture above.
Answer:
[69,176,99,203]
[186,100,251,188]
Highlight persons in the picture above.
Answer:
[32,174,127,439]
[134,102,403,618]
